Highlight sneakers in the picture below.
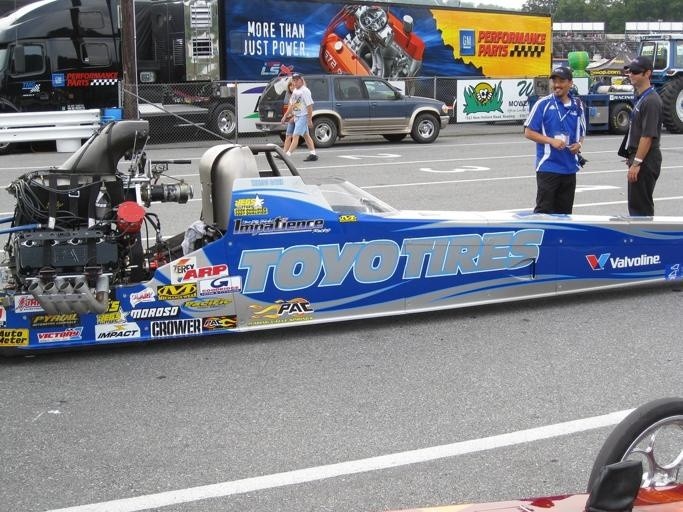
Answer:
[303,154,318,161]
[273,154,284,161]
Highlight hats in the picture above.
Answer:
[549,67,572,79]
[292,73,300,78]
[624,55,652,71]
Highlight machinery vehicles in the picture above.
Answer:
[575,33,683,140]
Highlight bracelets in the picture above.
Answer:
[576,141,582,148]
[631,156,643,167]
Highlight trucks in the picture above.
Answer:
[0,0,552,154]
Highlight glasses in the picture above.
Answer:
[628,69,649,74]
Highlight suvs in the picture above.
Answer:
[252,73,455,148]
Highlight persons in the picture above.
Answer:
[620,54,662,216]
[522,65,588,214]
[272,72,319,163]
[281,80,295,155]
